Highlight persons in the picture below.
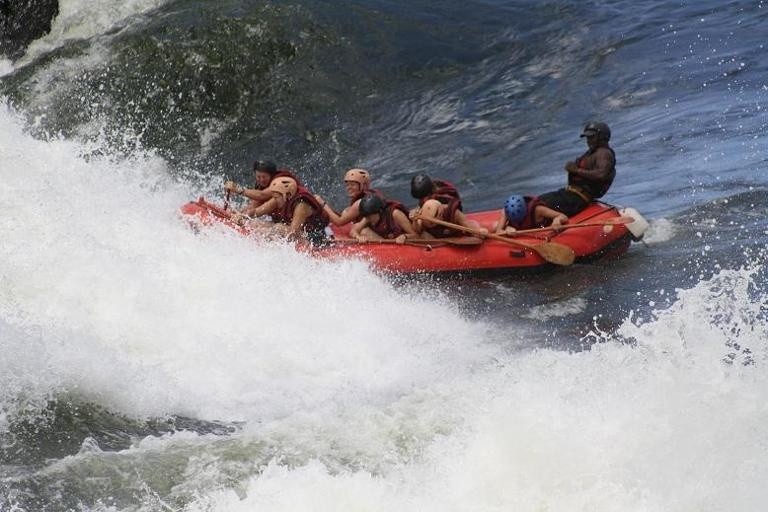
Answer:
[225,174,330,254]
[404,198,489,241]
[410,173,463,212]
[310,167,389,242]
[535,121,617,218]
[490,192,569,238]
[218,156,305,224]
[345,192,436,244]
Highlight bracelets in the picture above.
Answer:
[401,233,408,240]
[319,202,327,209]
[234,184,245,193]
[576,167,582,174]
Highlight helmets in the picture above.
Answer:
[343,168,372,194]
[578,121,612,144]
[268,177,299,202]
[252,155,279,177]
[358,194,381,216]
[409,172,433,200]
[503,193,528,227]
[418,199,443,229]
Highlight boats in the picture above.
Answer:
[180,197,650,281]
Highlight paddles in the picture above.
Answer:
[322,236,483,245]
[410,213,575,266]
[490,216,635,235]
